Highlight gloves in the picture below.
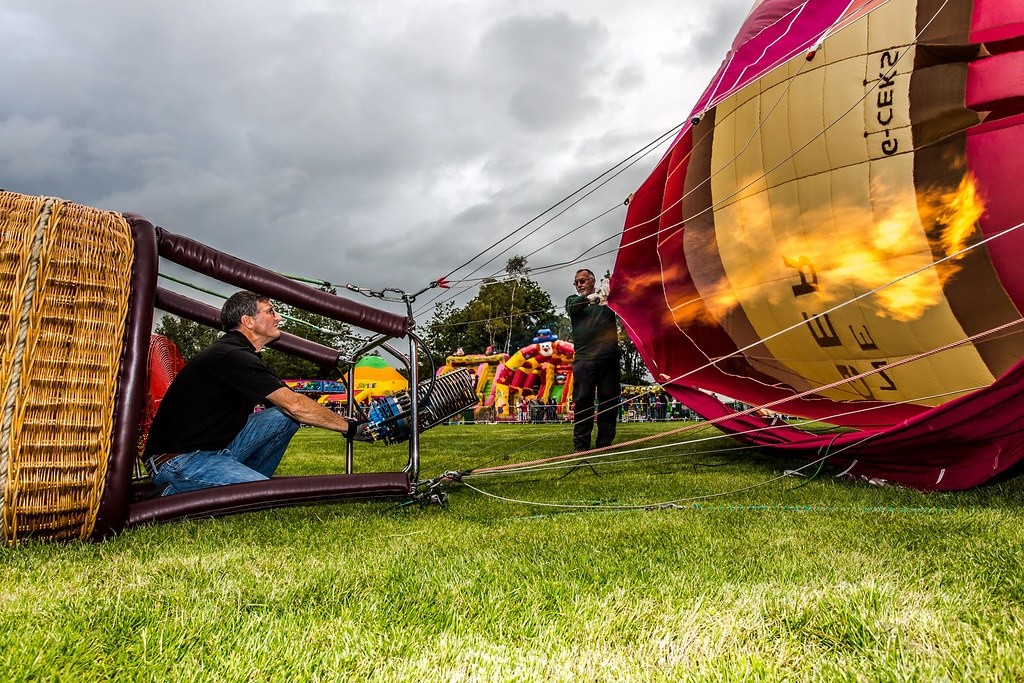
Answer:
[341,422,376,444]
[587,279,610,307]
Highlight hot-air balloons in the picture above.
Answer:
[0,1,1023,547]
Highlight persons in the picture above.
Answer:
[254,345,741,424]
[142,292,374,497]
[566,269,620,452]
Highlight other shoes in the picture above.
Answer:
[144,480,170,501]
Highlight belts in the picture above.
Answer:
[144,453,182,475]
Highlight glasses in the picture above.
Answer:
[251,307,278,315]
[573,278,593,286]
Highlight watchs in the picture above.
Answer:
[342,419,357,438]
